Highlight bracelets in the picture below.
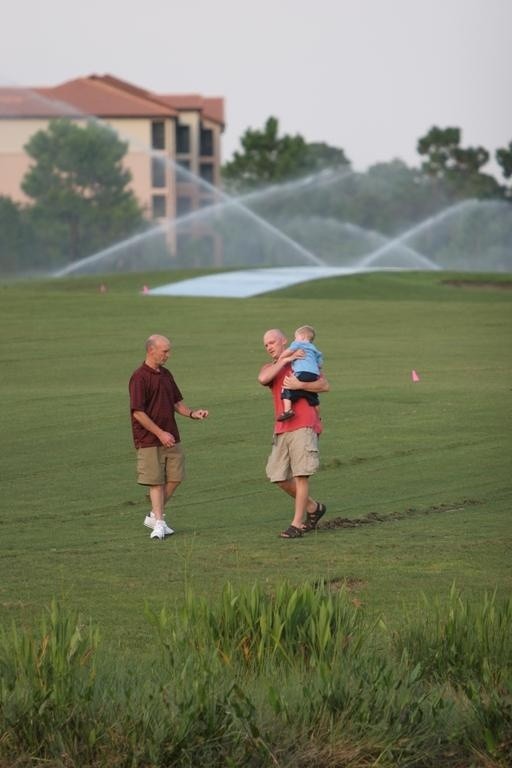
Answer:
[190,412,195,420]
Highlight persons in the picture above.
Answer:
[272,324,320,422]
[257,328,330,539]
[128,334,208,541]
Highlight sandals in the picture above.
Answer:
[280,526,304,538]
[276,409,295,421]
[302,503,326,531]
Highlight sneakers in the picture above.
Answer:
[143,512,174,535]
[150,524,165,540]
